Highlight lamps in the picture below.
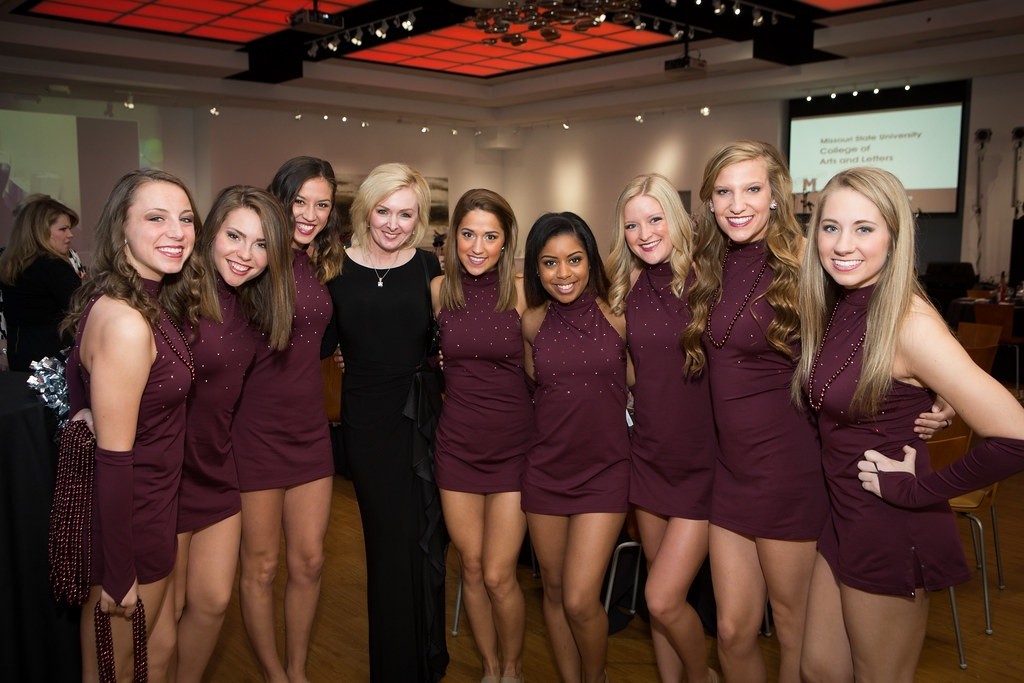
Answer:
[304,1,790,61]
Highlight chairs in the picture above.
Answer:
[918,322,1011,670]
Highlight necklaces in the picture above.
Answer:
[808,289,868,409]
[706,245,767,347]
[134,295,196,384]
[366,246,401,288]
[47,416,94,605]
[93,593,150,683]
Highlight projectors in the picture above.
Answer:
[665,56,707,73]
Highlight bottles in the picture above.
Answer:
[996,270,1009,304]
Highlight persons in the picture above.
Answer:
[231,157,332,683]
[679,139,956,683]
[320,163,452,683]
[601,170,720,683]
[67,181,295,683]
[436,210,636,683]
[789,167,1024,683]
[336,187,534,683]
[0,190,85,372]
[57,165,207,683]
